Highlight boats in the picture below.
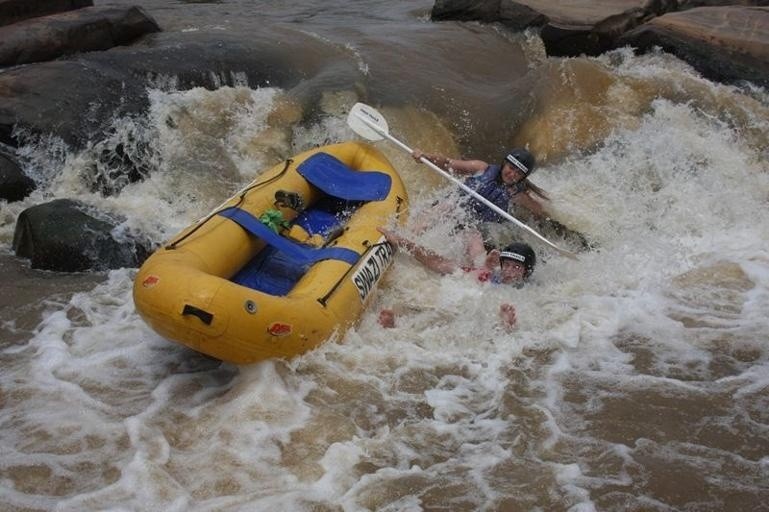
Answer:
[131,139,410,364]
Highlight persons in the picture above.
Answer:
[407,147,552,274]
[374,222,537,334]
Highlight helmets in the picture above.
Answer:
[498,149,535,187]
[499,243,536,279]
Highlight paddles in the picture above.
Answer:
[345,101,580,262]
